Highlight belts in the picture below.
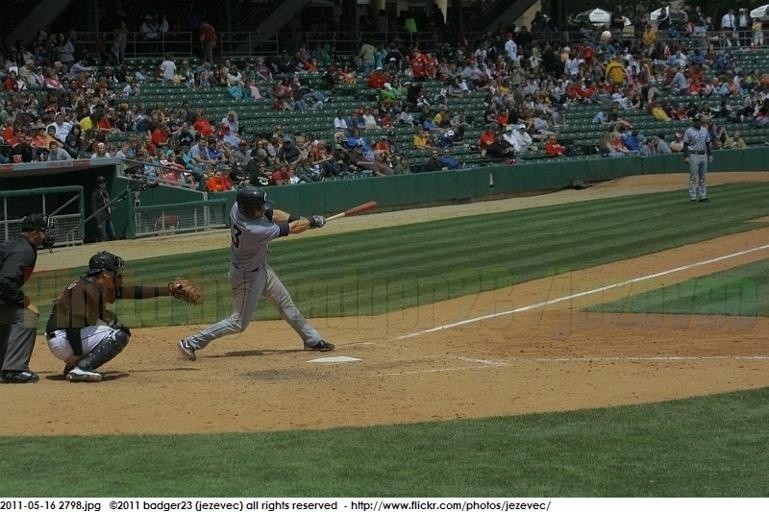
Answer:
[47,333,55,339]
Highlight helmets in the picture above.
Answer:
[22,213,57,248]
[87,254,124,287]
[238,187,267,212]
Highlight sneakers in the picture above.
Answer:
[304,340,333,351]
[179,340,196,361]
[64,364,102,381]
[0,371,39,383]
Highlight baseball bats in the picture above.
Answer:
[324,201,376,222]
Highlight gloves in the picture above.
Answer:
[308,215,324,228]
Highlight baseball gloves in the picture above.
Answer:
[169,276,204,304]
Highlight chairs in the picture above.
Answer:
[0,12,768,203]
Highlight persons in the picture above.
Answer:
[45,250,204,384]
[177,185,336,361]
[0,0,768,192]
[90,176,116,242]
[681,114,714,205]
[0,212,60,383]
